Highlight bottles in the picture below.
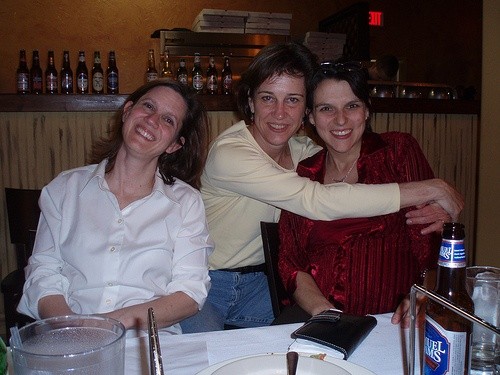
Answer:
[221,55,233,95]
[192,53,203,96]
[16,49,30,95]
[45,50,58,95]
[91,50,104,94]
[76,50,89,94]
[206,53,218,96]
[144,49,158,84]
[176,58,187,87]
[60,50,73,94]
[160,50,171,79]
[106,51,119,95]
[422,222,474,375]
[0,336,8,375]
[30,50,43,95]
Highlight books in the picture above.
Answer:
[288,308,378,361]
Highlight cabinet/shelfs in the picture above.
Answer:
[150,28,291,95]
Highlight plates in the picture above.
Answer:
[190,352,376,375]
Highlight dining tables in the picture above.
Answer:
[0,311,425,375]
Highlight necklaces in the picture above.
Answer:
[325,152,359,183]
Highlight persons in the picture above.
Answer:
[16,78,215,339]
[361,54,400,93]
[277,58,445,329]
[179,42,465,334]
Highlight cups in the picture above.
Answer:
[9,314,125,375]
[466,266,500,375]
